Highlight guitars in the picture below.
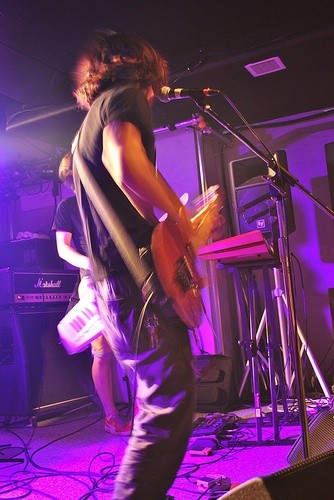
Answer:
[152,186,226,331]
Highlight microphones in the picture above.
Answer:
[9,413,47,426]
[158,86,219,102]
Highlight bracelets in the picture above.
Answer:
[165,204,184,226]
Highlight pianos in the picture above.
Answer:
[197,230,274,264]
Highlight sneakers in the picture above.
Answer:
[104,410,133,436]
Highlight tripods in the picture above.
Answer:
[238,266,334,404]
[117,374,134,417]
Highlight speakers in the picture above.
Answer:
[0,305,96,415]
[228,150,296,239]
[192,354,238,413]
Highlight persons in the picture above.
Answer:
[70,28,198,500]
[53,152,133,437]
